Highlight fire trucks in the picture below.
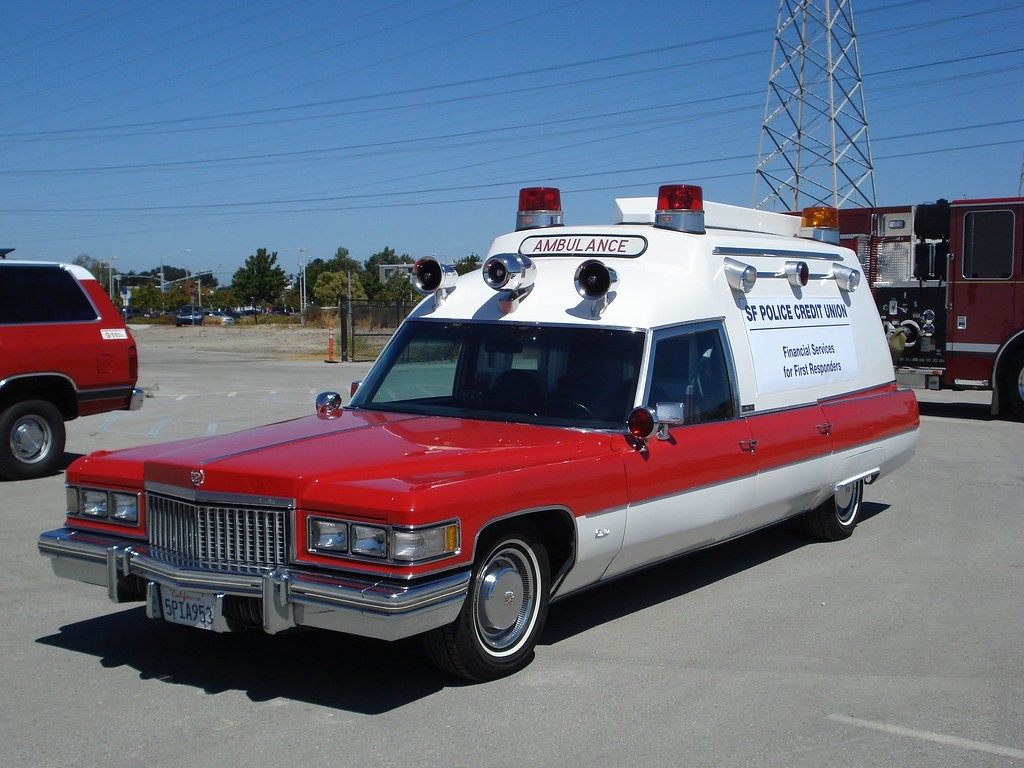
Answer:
[777,197,1024,421]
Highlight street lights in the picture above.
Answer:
[159,249,191,315]
[99,254,118,287]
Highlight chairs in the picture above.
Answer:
[478,368,546,416]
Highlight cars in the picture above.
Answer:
[204,310,235,326]
[175,306,205,326]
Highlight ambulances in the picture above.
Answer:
[34,183,925,682]
[0,247,145,481]
